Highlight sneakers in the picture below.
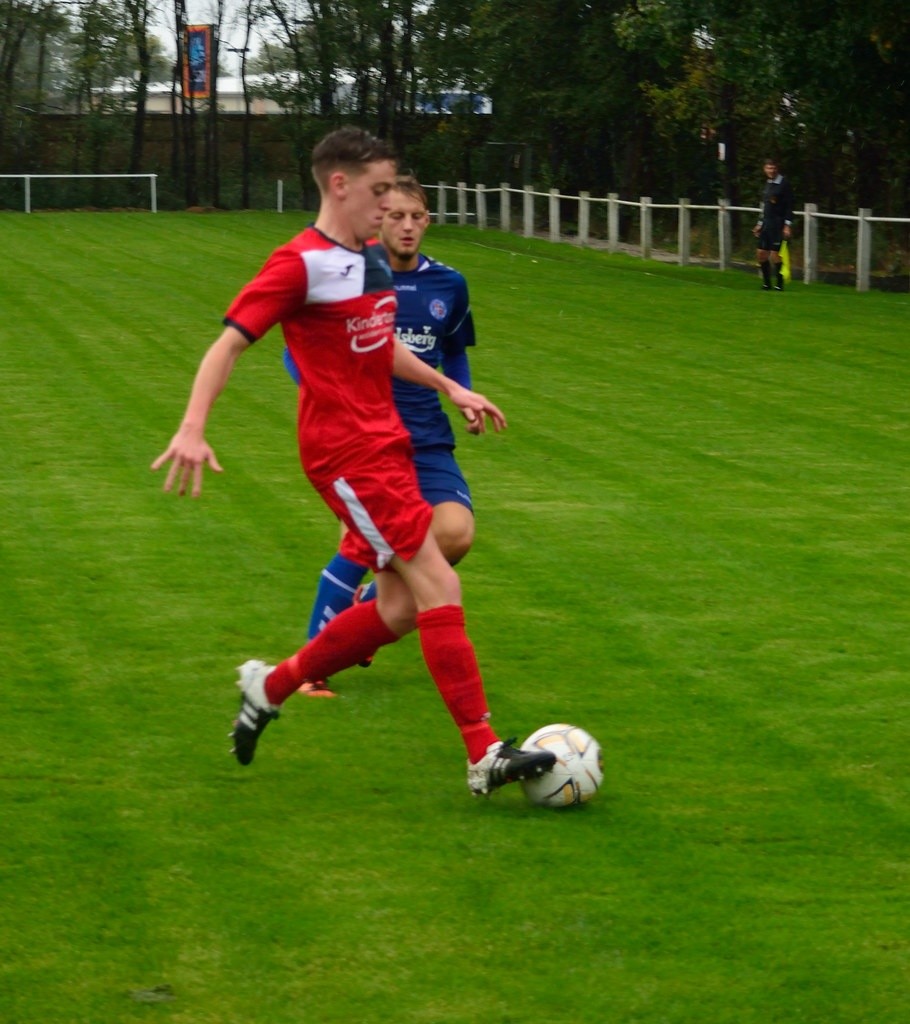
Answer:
[760,283,772,290]
[229,661,275,765]
[774,285,783,291]
[354,583,374,672]
[467,740,560,797]
[295,675,341,702]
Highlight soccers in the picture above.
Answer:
[515,722,605,808]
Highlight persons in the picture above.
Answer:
[752,158,794,291]
[287,171,477,698]
[149,125,554,796]
